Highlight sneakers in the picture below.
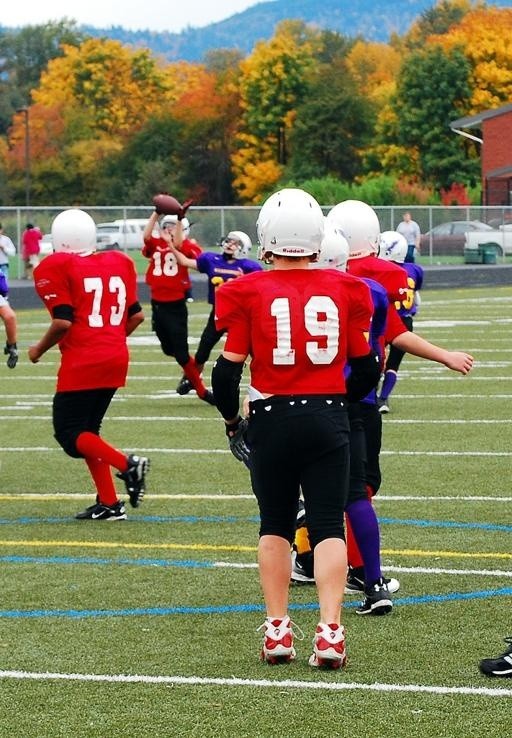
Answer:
[73,494,128,522]
[255,614,305,666]
[115,453,150,508]
[377,397,391,414]
[289,544,316,587]
[308,622,350,670]
[176,375,194,396]
[196,388,217,406]
[342,564,400,596]
[355,577,394,617]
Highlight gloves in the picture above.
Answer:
[154,191,173,215]
[222,413,252,462]
[4,341,19,369]
[178,198,194,219]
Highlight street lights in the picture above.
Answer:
[16,108,31,279]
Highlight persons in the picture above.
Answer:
[0,222,16,304]
[22,224,43,270]
[29,208,149,523]
[0,292,19,369]
[142,187,474,670]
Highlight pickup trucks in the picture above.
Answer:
[465,225,512,258]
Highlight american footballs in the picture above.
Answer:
[151,194,181,214]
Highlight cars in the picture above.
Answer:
[420,221,497,254]
[96,217,160,250]
[37,233,52,253]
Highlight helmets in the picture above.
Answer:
[51,208,98,258]
[325,199,382,260]
[221,231,253,261]
[256,186,326,261]
[313,226,349,270]
[374,230,409,265]
[158,214,191,240]
